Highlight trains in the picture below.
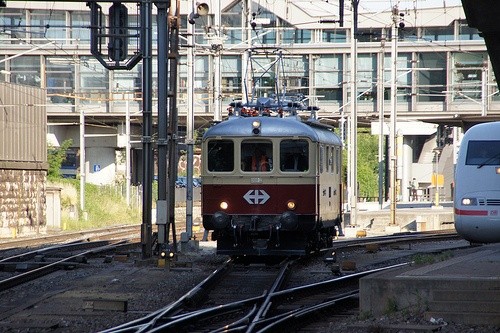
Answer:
[200,97,344,261]
[453,121,500,246]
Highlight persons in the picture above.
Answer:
[246,146,270,172]
[409,176,418,200]
[283,146,309,172]
